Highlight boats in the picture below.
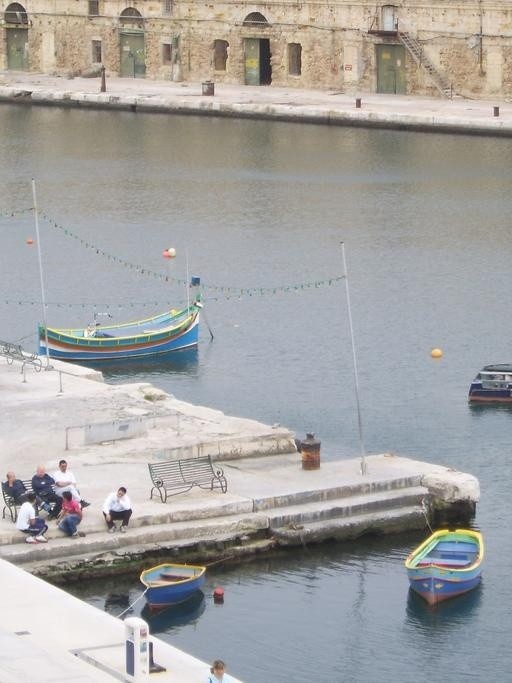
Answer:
[141,589,205,638]
[403,527,484,603]
[36,292,203,360]
[405,579,485,632]
[468,362,512,403]
[66,342,200,382]
[138,561,207,608]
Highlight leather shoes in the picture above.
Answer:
[43,503,63,521]
[81,501,91,507]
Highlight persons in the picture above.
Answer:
[102,487,133,533]
[84,322,106,338]
[207,659,230,683]
[5,460,91,543]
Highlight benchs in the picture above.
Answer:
[147,454,227,503]
[1,478,80,523]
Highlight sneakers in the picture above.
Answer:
[26,535,47,542]
[108,526,127,533]
[72,531,86,538]
